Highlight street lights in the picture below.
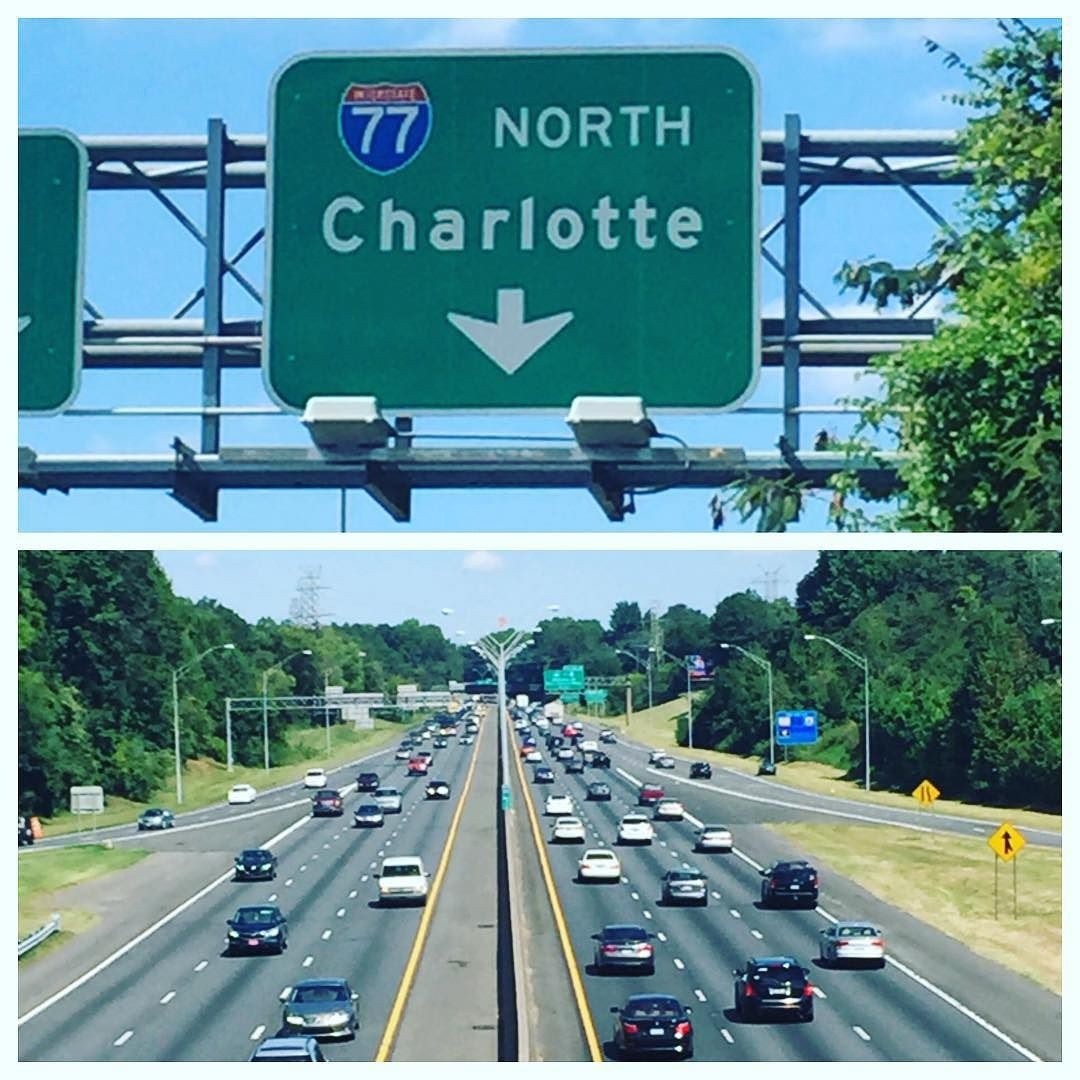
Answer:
[264,648,313,778]
[803,633,872,792]
[439,603,561,787]
[1041,618,1063,626]
[647,645,695,752]
[323,651,365,757]
[615,648,653,725]
[720,643,777,761]
[172,642,234,806]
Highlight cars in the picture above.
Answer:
[280,976,361,1042]
[233,848,279,881]
[693,824,737,851]
[137,808,176,831]
[226,784,258,805]
[247,1036,333,1061]
[19,816,33,845]
[226,905,288,954]
[578,848,623,883]
[590,924,657,973]
[817,922,888,961]
[658,868,709,907]
[303,693,711,844]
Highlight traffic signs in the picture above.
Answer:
[264,46,762,408]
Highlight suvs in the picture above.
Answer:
[757,857,818,910]
[731,956,816,1022]
[373,856,433,905]
[610,995,696,1059]
